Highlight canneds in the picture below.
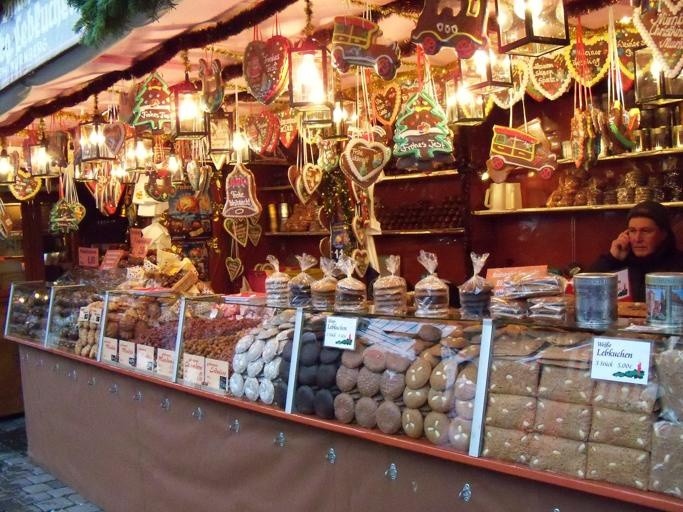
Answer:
[645,272,683,328]
[573,272,618,331]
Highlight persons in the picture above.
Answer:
[587,201,683,301]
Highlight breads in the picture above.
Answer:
[229,308,296,409]
[289,277,311,306]
[481,322,683,498]
[104,293,181,344]
[9,289,49,342]
[296,309,482,450]
[546,167,604,207]
[458,283,495,317]
[50,289,98,347]
[265,275,289,307]
[73,300,103,357]
[373,277,407,316]
[603,160,683,202]
[413,282,450,317]
[334,278,369,312]
[311,276,337,311]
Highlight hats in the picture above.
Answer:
[626,201,675,251]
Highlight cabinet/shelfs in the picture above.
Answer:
[3,279,682,511]
